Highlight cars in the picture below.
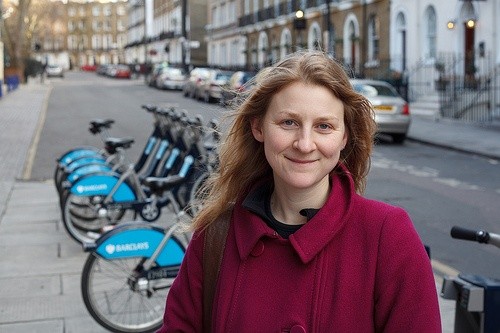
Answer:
[46,64,64,77]
[349,80,412,145]
[156,68,184,89]
[220,71,256,107]
[184,68,235,103]
[82,64,131,78]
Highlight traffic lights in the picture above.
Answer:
[294,10,307,29]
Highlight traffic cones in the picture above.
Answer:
[53,104,221,244]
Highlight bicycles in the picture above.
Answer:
[449,224,499,250]
[81,175,191,333]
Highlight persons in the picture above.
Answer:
[162,38,443,333]
